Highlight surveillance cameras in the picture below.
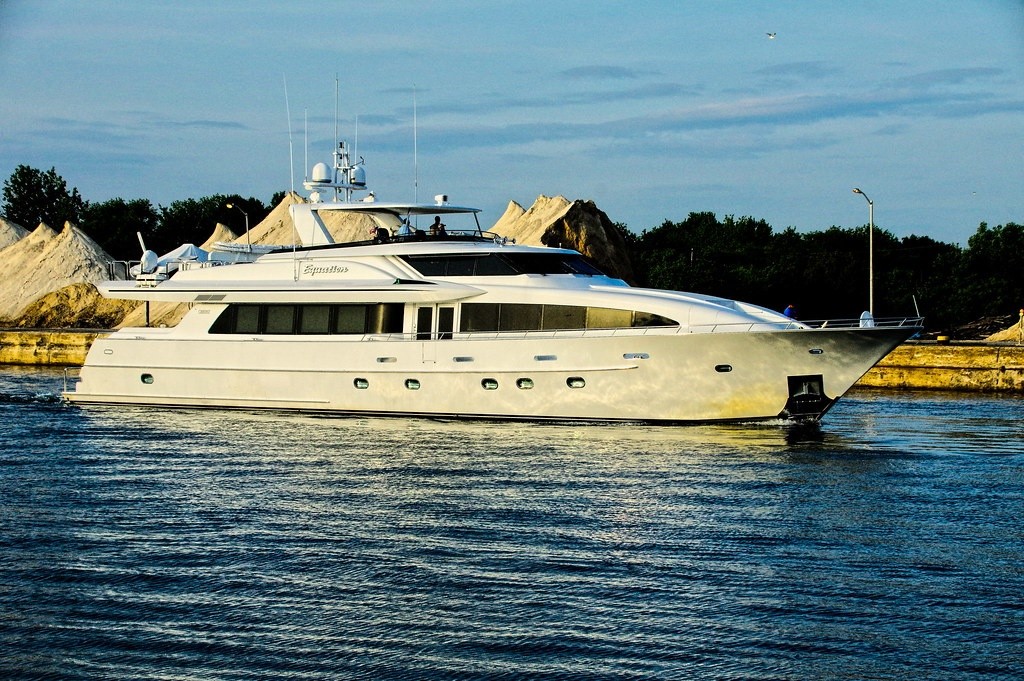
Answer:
[435,195,448,201]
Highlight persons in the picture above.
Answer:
[370,226,389,244]
[398,218,412,234]
[430,216,445,235]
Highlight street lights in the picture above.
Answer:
[853,189,873,325]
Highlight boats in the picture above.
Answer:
[55,74,925,422]
